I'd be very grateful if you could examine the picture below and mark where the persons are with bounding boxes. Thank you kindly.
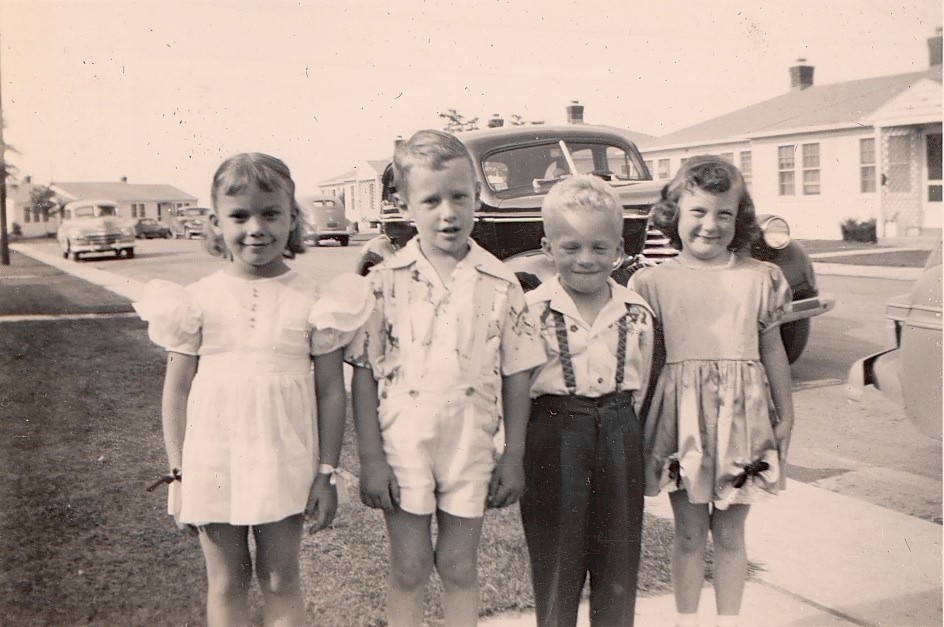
[628,156,793,627]
[146,153,375,627]
[342,129,549,627]
[518,175,656,627]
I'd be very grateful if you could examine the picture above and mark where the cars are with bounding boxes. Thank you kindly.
[133,217,173,241]
[846,232,943,441]
[174,206,214,241]
[352,121,836,367]
[295,194,349,247]
[55,200,136,260]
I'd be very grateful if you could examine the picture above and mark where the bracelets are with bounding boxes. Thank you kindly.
[146,468,182,492]
[318,464,349,485]
